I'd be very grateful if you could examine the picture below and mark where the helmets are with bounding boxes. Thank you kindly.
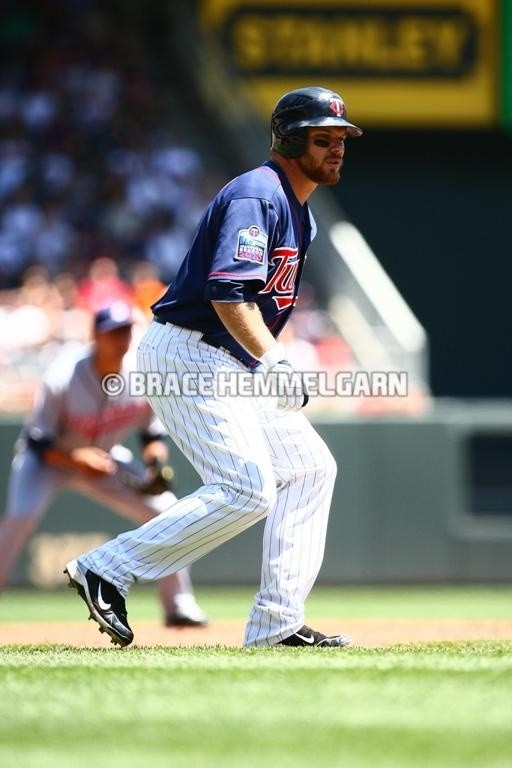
[265,89,366,158]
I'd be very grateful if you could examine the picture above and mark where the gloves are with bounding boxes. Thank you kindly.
[261,342,309,412]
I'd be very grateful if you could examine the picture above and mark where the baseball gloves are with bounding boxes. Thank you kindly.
[131,457,174,495]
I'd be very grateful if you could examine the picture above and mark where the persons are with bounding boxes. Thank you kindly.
[1,300,211,628]
[0,0,432,418]
[61,86,363,651]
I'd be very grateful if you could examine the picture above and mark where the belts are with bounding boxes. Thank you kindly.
[153,313,255,372]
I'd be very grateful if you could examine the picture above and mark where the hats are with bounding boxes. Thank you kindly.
[85,303,136,338]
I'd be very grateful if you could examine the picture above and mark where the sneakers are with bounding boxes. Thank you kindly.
[63,559,133,647]
[273,625,354,646]
[160,610,214,629]
